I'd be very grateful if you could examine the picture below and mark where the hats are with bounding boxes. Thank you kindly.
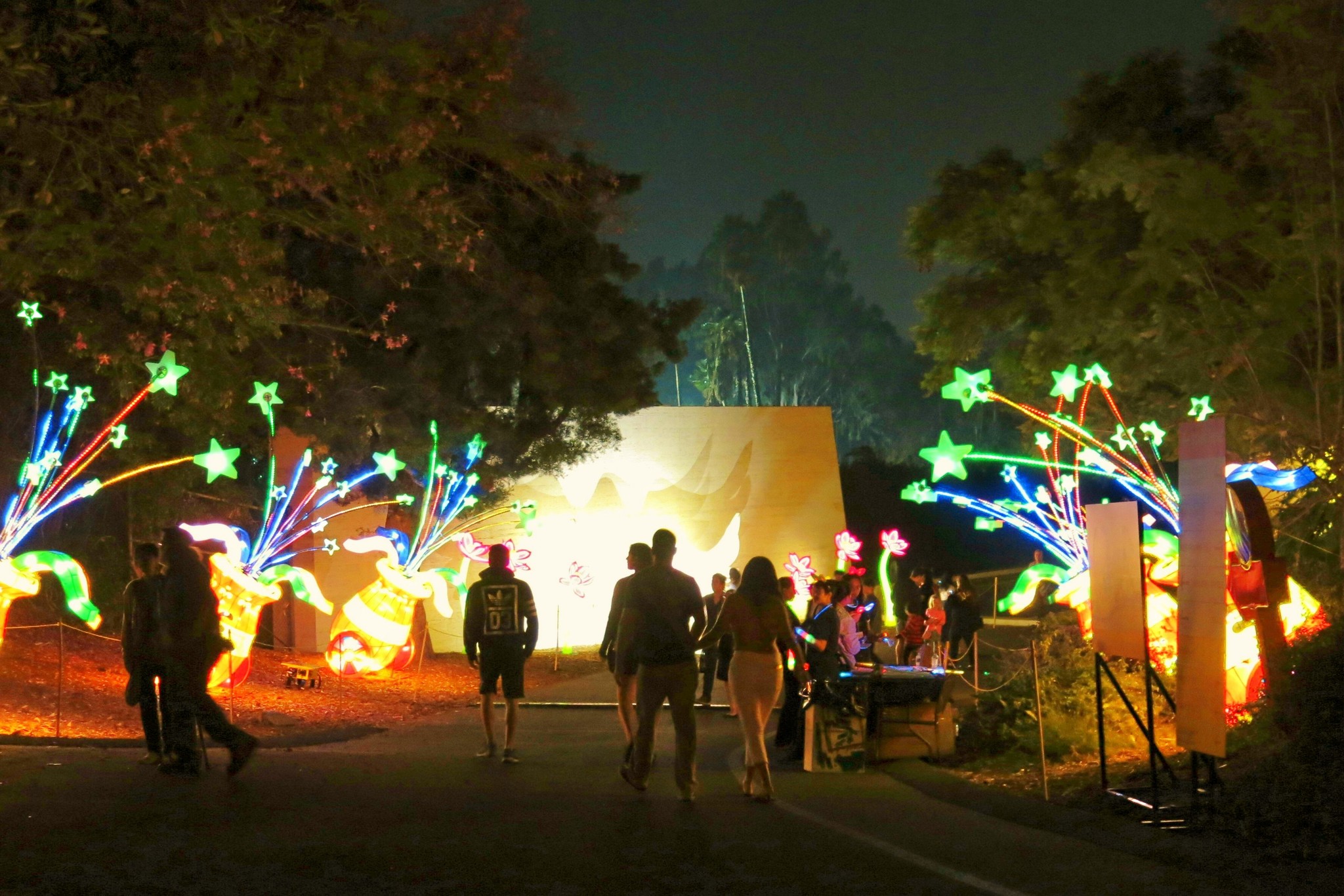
[161,526,195,545]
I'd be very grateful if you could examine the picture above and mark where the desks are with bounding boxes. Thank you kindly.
[840,667,964,760]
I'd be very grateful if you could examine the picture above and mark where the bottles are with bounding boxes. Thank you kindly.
[931,653,939,668]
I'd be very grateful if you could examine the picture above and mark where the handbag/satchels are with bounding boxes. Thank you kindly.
[125,675,143,706]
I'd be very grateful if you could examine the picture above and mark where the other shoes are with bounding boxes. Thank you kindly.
[695,696,712,706]
[226,731,258,777]
[158,749,203,775]
[138,751,157,765]
[618,764,648,790]
[502,748,519,764]
[623,742,633,763]
[476,743,496,757]
[778,746,804,764]
[678,794,695,804]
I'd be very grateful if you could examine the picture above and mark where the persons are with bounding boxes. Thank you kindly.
[599,528,1046,807]
[122,529,258,774]
[463,544,538,762]
